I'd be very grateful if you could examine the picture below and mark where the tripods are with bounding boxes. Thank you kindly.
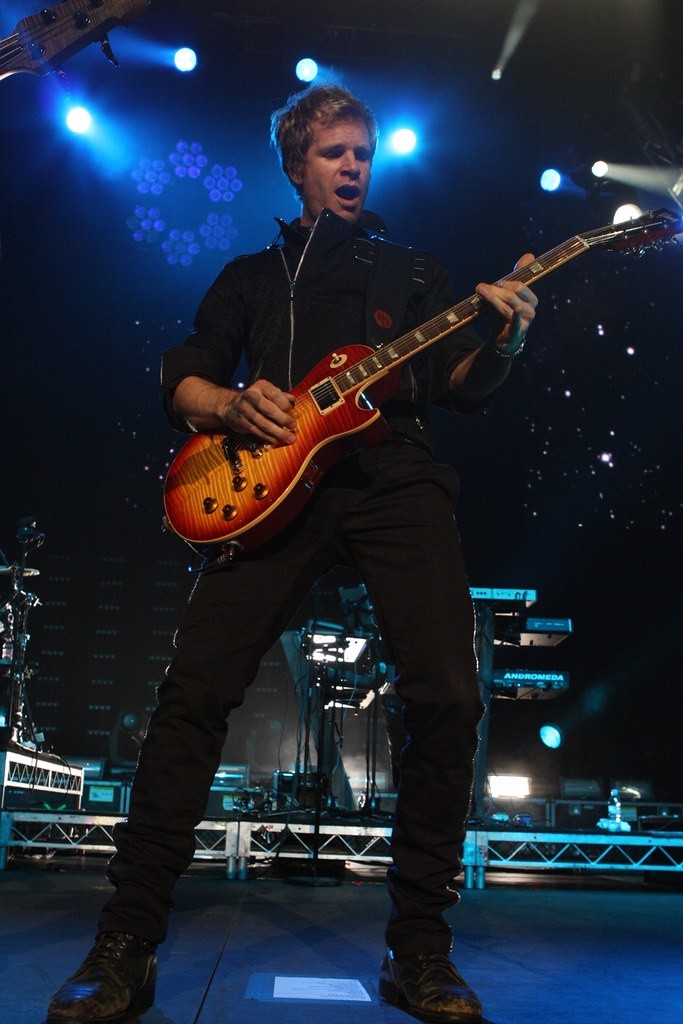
[258,654,397,825]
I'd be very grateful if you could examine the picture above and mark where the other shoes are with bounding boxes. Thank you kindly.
[379,946,483,1022]
[47,933,157,1024]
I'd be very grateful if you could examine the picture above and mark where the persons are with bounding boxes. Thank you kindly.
[45,81,543,1024]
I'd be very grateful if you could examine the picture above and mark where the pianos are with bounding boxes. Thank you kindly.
[492,615,573,648]
[490,668,569,700]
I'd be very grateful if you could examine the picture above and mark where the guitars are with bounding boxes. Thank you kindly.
[162,207,683,559]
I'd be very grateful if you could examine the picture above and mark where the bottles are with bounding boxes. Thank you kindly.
[607,789,621,822]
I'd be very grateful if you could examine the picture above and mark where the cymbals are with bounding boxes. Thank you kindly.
[0,566,40,577]
